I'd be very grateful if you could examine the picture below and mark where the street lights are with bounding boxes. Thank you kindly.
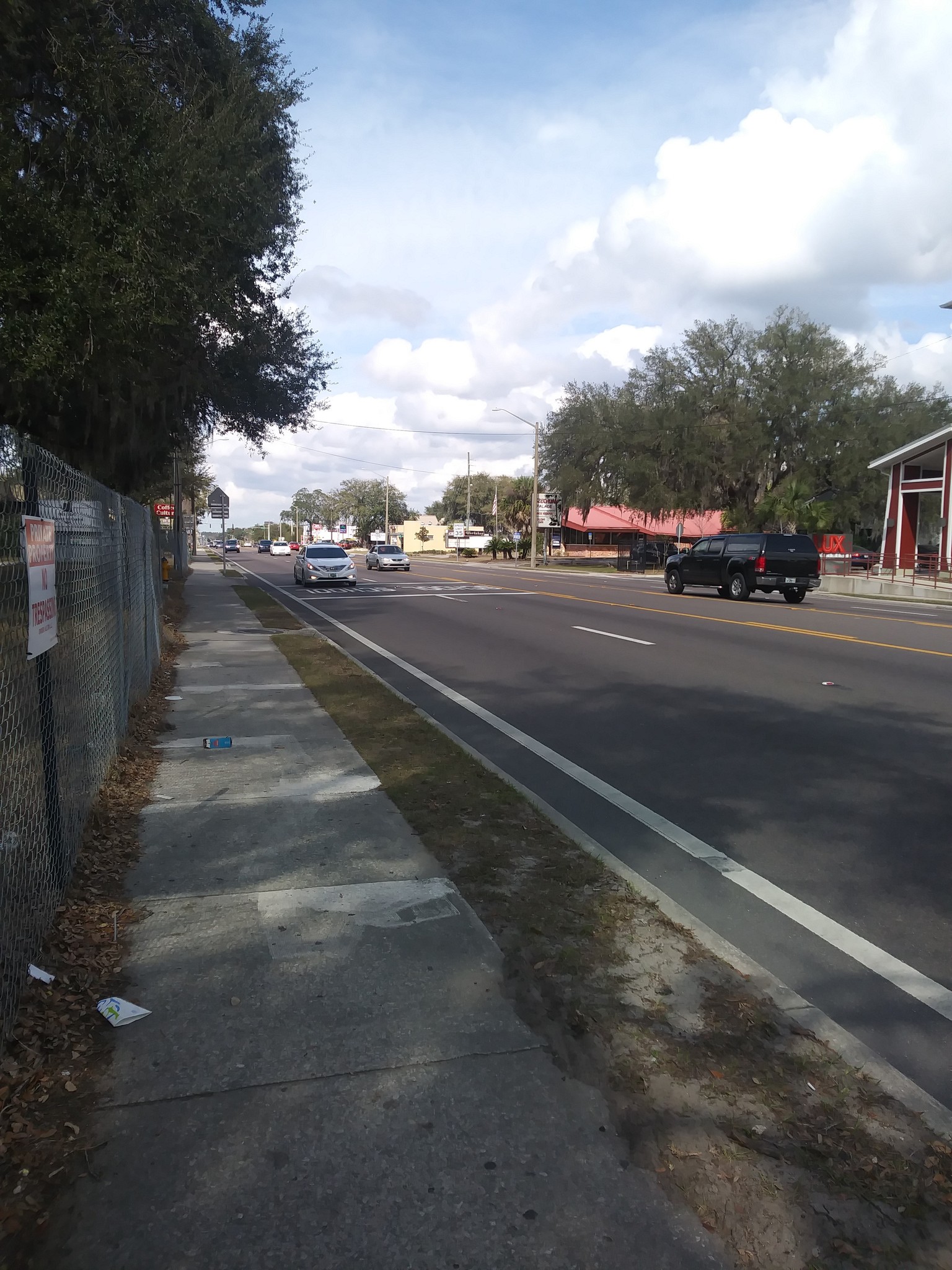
[282,503,298,543]
[492,408,539,567]
[361,468,389,545]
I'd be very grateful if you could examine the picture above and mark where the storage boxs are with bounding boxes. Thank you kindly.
[203,737,233,749]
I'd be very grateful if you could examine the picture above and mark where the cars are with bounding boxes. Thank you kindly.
[244,542,252,547]
[294,544,357,587]
[917,544,939,568]
[348,540,358,547]
[216,541,226,549]
[209,542,213,547]
[270,542,291,556]
[339,540,350,549]
[852,545,878,570]
[303,541,316,545]
[365,544,410,571]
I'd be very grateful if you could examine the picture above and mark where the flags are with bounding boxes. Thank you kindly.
[493,493,498,516]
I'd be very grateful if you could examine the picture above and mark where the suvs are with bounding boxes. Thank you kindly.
[288,542,299,551]
[225,540,240,553]
[631,541,682,565]
[213,540,220,548]
[664,533,822,603]
[257,540,272,553]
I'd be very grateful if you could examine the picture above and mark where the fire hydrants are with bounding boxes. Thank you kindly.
[162,557,170,581]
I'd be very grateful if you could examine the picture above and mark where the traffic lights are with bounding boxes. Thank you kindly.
[252,529,254,532]
[232,529,234,532]
[243,528,244,532]
